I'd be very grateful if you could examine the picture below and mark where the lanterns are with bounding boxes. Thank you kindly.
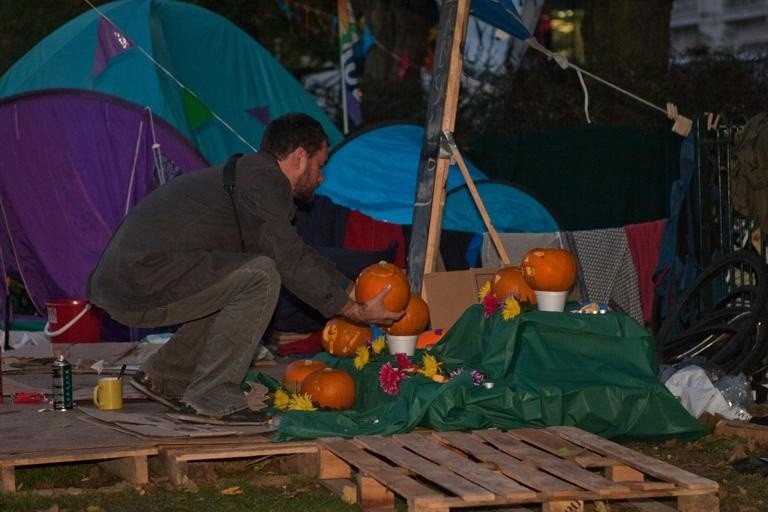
[284,358,327,396]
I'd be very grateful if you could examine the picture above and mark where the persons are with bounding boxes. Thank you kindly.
[80,108,408,428]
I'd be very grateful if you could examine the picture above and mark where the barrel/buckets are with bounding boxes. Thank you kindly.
[43,296,105,343]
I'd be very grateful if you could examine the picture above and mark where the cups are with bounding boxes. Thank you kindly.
[92,377,123,410]
[386,333,418,356]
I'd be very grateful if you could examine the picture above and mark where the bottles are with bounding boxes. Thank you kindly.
[52,353,73,409]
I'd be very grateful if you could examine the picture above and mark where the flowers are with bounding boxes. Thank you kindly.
[354,337,487,399]
[473,278,537,322]
[268,382,320,413]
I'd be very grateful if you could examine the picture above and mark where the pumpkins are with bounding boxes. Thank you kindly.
[284,357,326,387]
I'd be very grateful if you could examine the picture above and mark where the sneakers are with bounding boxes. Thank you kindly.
[130,368,181,413]
[180,403,268,429]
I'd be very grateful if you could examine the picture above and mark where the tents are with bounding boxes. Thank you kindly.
[0,1,564,344]
